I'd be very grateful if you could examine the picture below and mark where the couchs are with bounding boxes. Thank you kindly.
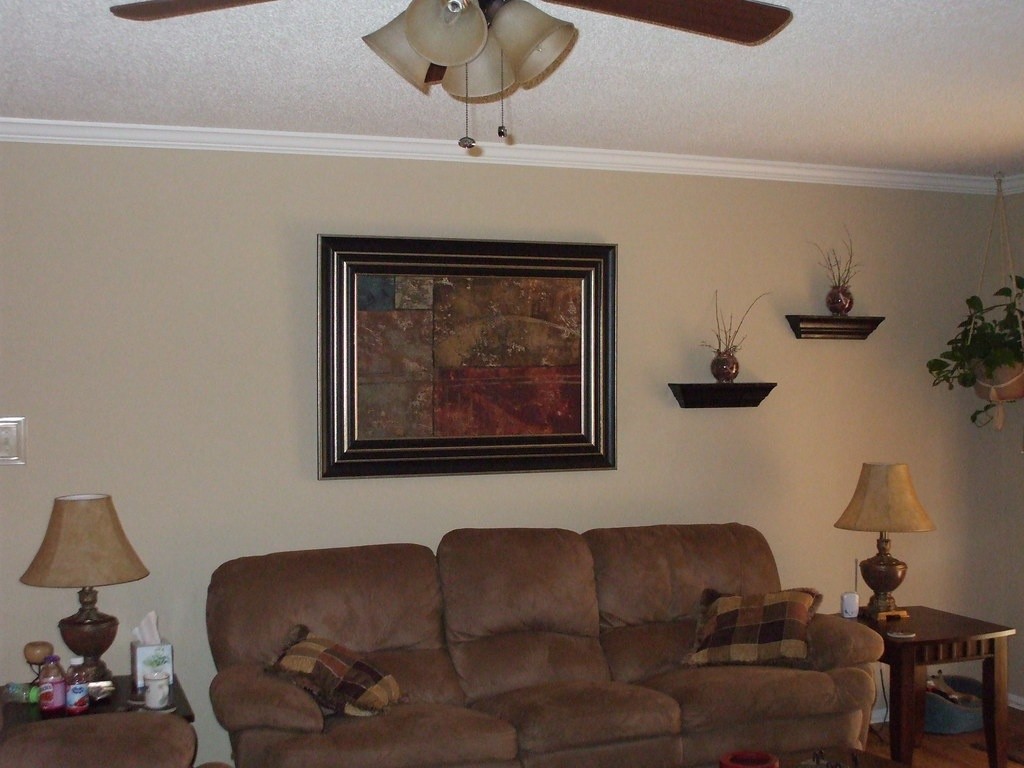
[0,714,196,768]
[205,521,884,768]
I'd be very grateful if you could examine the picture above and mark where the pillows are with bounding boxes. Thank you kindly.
[685,586,822,669]
[264,625,400,717]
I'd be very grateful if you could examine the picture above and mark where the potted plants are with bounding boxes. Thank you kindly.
[926,274,1024,431]
[803,225,861,316]
[700,286,771,383]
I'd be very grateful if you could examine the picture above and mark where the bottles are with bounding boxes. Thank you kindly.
[63,656,89,716]
[2,681,40,705]
[38,656,65,719]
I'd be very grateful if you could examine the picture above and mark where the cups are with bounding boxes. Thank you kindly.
[143,671,171,710]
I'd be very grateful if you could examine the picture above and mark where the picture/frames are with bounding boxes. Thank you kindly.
[316,232,618,481]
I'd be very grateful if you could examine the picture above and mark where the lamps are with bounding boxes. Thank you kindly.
[832,462,937,617]
[359,0,580,150]
[18,493,150,656]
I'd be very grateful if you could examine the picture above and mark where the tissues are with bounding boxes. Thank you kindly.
[130,608,175,688]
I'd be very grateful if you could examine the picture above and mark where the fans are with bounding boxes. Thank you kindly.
[110,0,793,47]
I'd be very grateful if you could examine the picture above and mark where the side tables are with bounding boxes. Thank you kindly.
[0,674,194,722]
[831,605,1017,768]
